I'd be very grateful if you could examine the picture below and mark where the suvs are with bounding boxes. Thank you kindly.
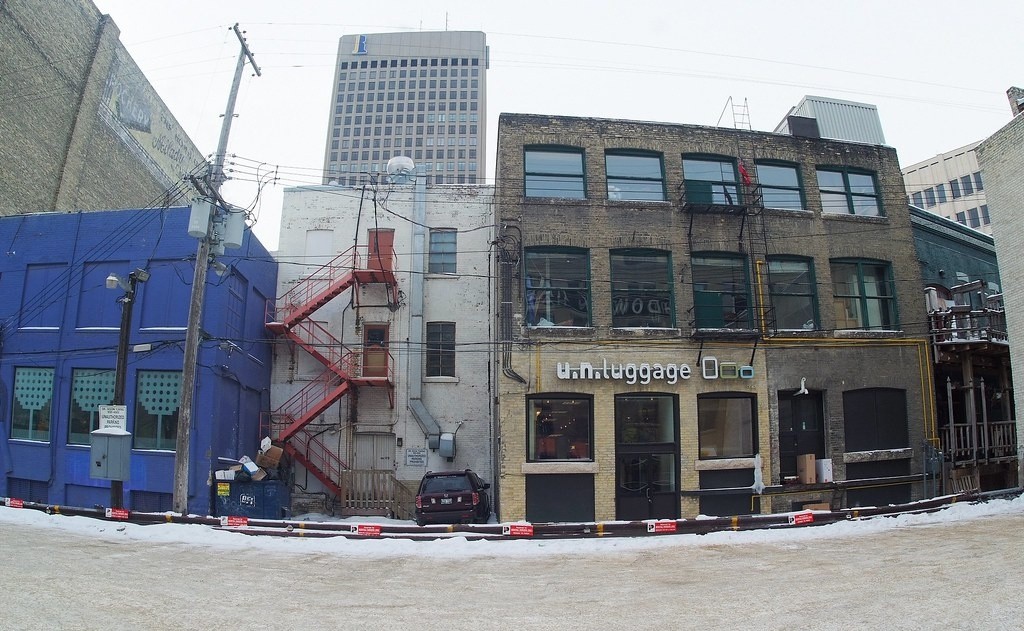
[415,468,491,526]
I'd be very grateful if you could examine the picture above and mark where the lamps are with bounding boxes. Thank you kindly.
[397,437,402,446]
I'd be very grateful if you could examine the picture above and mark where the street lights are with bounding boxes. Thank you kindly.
[105,273,133,509]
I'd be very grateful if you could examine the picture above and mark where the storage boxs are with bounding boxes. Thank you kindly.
[796,453,815,484]
[802,502,830,509]
[256,445,283,468]
[815,458,832,483]
[218,457,238,468]
[250,468,266,480]
[230,465,242,471]
[244,462,258,475]
[240,455,252,463]
[215,470,235,480]
